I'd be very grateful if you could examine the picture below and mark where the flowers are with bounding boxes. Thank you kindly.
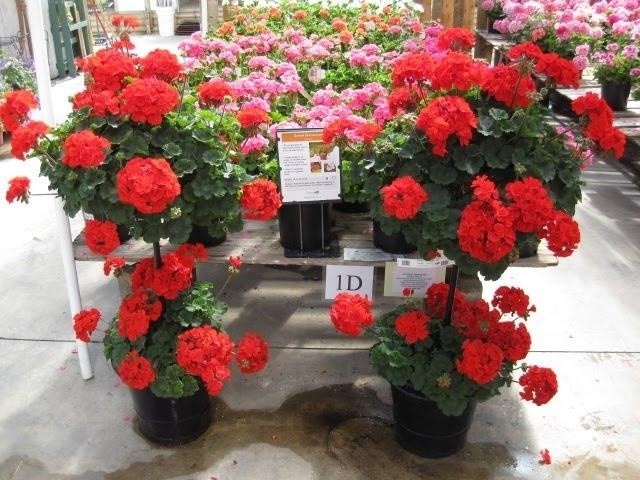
[557,91,626,169]
[181,0,477,221]
[1,47,182,255]
[482,1,640,81]
[110,16,137,50]
[72,242,269,398]
[457,171,582,265]
[478,41,581,110]
[328,286,558,406]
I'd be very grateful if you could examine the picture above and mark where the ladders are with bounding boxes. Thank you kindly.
[48,0,93,80]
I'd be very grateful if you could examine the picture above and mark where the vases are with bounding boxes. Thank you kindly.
[391,380,475,459]
[130,373,212,445]
